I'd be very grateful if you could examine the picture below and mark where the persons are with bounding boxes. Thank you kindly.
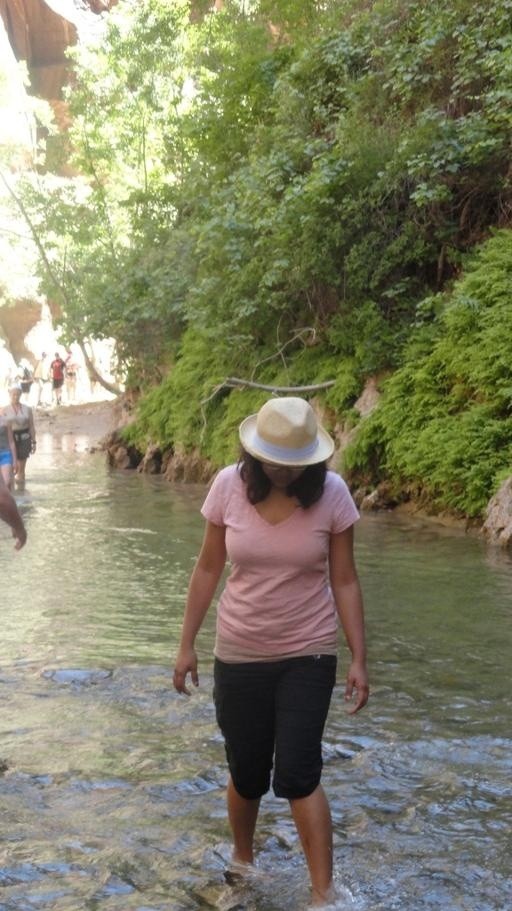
[32,350,49,407]
[0,413,18,490]
[47,353,69,406]
[0,467,28,551]
[64,356,79,401]
[0,383,38,484]
[172,394,371,911]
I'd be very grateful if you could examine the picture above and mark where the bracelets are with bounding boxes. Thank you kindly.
[30,440,36,444]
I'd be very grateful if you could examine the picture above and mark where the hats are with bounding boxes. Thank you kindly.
[238,394,336,466]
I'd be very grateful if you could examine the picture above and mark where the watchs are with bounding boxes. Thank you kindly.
[17,364,31,398]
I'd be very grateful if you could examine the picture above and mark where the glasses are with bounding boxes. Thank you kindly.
[261,463,308,471]
